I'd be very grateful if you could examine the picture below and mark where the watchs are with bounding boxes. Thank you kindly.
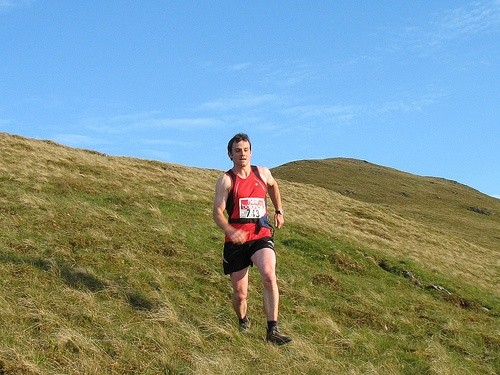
[275,209,284,215]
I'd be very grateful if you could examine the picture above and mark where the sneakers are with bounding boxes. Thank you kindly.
[239,318,248,328]
[266,329,291,345]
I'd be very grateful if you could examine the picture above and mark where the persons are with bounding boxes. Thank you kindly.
[213,132,293,346]
